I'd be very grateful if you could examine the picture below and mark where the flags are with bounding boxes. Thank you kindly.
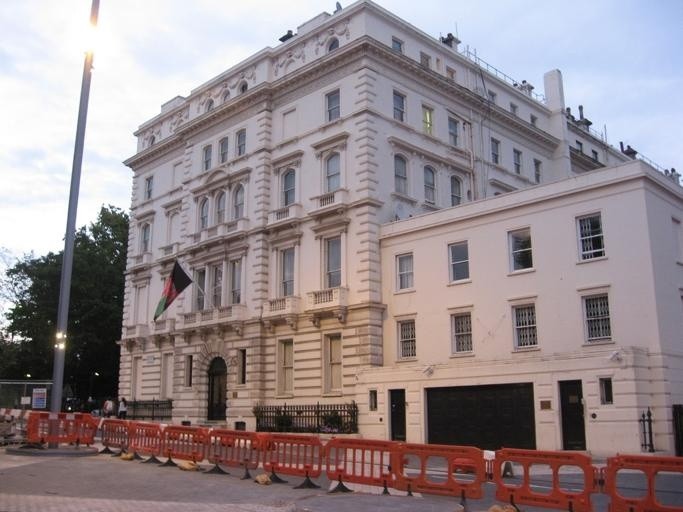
[152,257,193,322]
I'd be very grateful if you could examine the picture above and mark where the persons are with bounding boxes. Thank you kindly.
[117,396,128,420]
[103,396,114,418]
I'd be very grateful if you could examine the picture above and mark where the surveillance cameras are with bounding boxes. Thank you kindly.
[609,350,620,362]
[423,366,433,373]
